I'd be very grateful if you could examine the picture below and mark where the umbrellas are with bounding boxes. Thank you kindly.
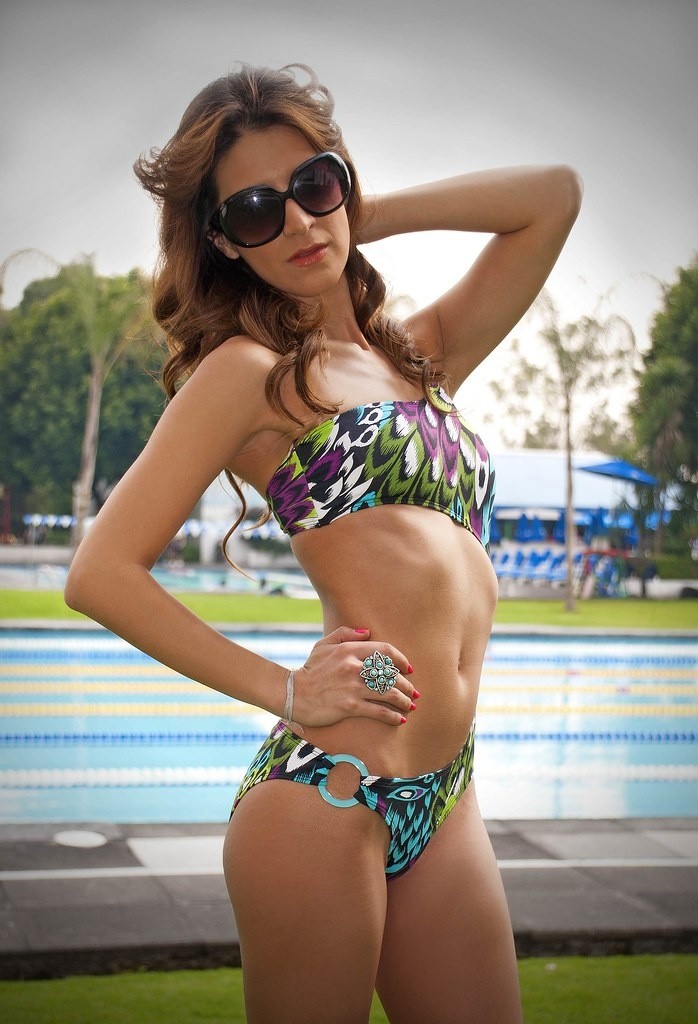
[489,512,607,545]
[577,461,657,486]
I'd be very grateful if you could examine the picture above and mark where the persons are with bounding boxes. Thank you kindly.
[64,64,582,1022]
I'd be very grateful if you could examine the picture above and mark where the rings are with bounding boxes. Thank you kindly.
[361,650,400,693]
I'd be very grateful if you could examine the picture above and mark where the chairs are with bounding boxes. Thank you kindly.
[489,549,657,599]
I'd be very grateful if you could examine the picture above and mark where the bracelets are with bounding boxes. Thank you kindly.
[284,668,294,720]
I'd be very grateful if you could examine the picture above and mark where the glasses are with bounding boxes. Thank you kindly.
[207,152,352,248]
